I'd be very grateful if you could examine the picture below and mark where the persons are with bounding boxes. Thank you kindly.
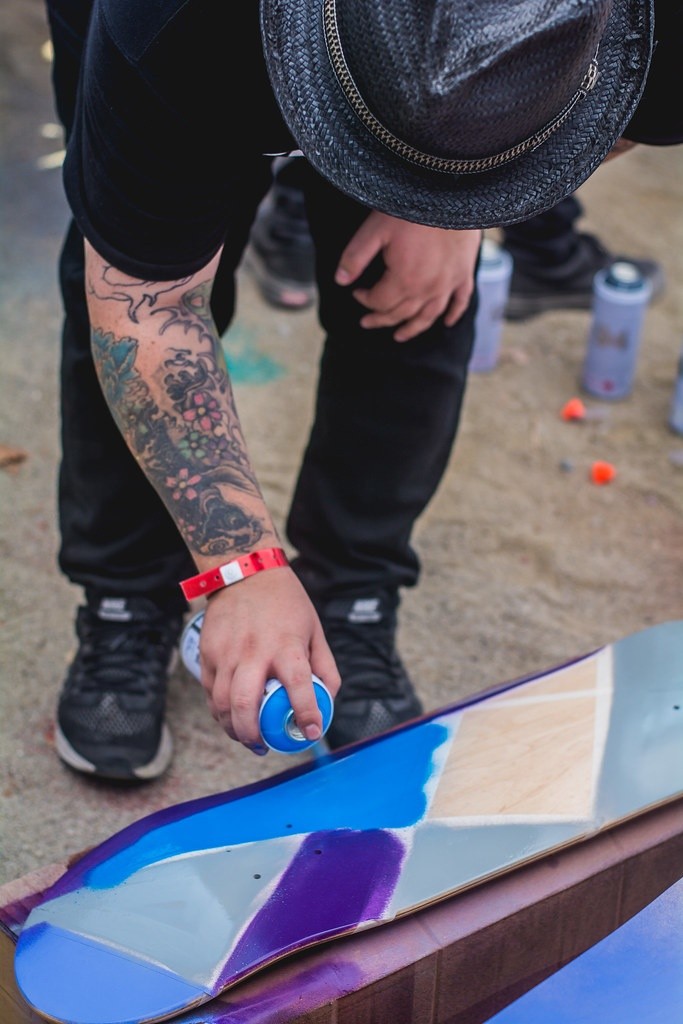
[245,0,682,320]
[44,1,655,793]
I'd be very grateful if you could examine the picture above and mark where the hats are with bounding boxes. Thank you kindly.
[260,0,655,231]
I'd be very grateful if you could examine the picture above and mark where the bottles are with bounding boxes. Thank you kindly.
[180,608,335,755]
[579,261,652,397]
[466,238,514,372]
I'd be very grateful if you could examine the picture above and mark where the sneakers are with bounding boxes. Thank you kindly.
[247,208,316,307]
[311,598,423,754]
[52,595,184,786]
[501,236,663,316]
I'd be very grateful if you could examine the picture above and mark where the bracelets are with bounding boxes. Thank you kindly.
[179,546,289,602]
